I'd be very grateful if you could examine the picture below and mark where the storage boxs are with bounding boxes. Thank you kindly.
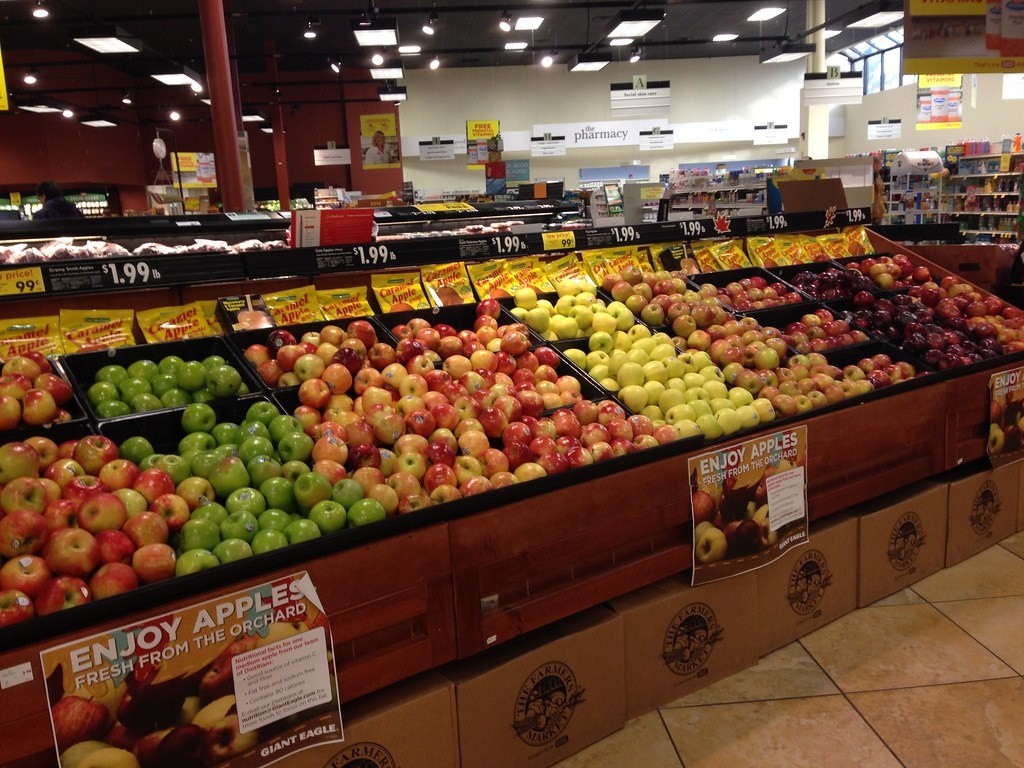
[0,242,1024,768]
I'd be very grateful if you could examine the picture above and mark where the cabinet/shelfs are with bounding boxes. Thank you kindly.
[595,152,1024,238]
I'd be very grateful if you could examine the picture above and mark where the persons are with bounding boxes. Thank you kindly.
[31,181,86,219]
[366,131,393,165]
[872,156,887,225]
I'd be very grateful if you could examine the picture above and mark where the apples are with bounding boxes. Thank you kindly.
[52,634,260,768]
[0,252,1023,627]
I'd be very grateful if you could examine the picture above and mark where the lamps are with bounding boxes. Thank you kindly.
[241,0,905,134]
[16,25,203,133]
